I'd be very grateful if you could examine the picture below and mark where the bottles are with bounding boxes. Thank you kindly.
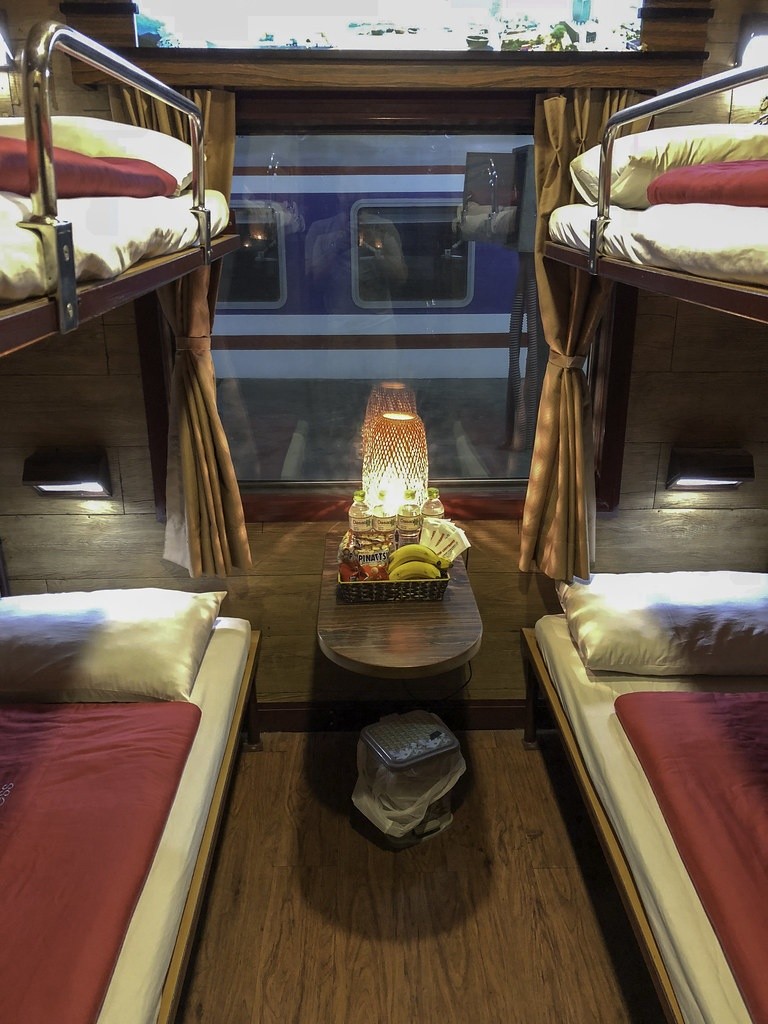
[373,490,397,553]
[422,488,445,526]
[349,491,372,538]
[397,489,421,548]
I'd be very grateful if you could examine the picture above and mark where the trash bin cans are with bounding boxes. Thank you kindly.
[358,709,460,848]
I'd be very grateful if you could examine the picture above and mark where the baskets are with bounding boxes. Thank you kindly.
[337,569,450,602]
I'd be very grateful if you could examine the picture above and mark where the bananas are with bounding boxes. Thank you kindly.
[388,544,453,580]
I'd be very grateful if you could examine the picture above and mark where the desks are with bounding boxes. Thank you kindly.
[316,531,483,679]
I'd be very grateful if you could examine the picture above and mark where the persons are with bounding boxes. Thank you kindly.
[304,173,408,423]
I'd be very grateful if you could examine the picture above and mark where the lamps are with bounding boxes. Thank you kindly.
[22,443,112,498]
[666,443,756,491]
[359,382,416,460]
[736,12,768,65]
[361,413,430,513]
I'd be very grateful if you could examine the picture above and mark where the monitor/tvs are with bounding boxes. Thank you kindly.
[130,0,647,57]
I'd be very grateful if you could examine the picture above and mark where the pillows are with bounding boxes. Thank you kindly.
[0,587,228,704]
[556,570,768,676]
[570,122,768,210]
[0,116,207,195]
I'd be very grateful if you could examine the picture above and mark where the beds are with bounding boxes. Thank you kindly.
[1,617,263,1024]
[0,21,242,356]
[544,63,768,327]
[519,613,768,1024]
